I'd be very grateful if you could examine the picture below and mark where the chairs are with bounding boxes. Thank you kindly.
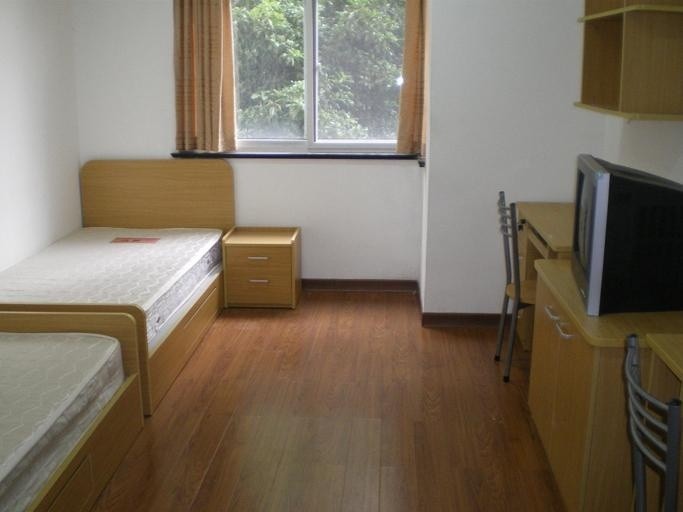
[624,334,682,512]
[493,191,538,383]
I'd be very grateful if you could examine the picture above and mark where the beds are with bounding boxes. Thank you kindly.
[0,311,144,512]
[0,158,235,417]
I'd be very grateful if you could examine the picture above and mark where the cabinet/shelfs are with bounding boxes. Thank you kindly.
[573,0,683,121]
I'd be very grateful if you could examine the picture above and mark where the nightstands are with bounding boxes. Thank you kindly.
[222,225,301,310]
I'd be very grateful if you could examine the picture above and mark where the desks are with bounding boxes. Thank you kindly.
[526,259,683,512]
[515,200,575,354]
[632,332,683,512]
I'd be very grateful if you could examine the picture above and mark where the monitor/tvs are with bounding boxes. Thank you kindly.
[570,152,683,318]
